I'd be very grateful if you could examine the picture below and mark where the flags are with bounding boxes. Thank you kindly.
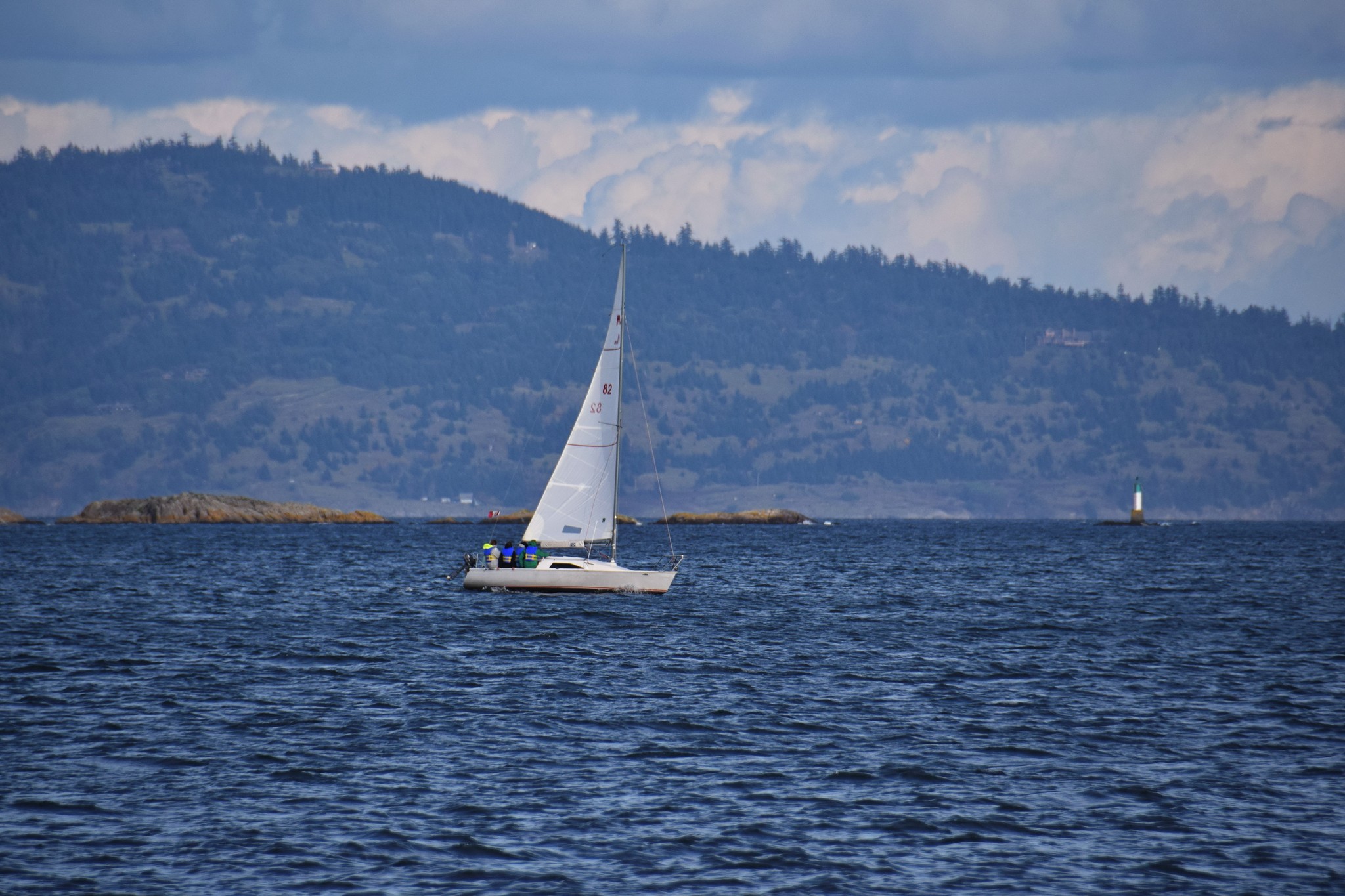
[488,511,501,518]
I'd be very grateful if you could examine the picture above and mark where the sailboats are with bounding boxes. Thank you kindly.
[447,242,681,595]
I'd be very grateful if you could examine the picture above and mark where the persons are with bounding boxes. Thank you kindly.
[519,539,551,568]
[483,539,501,569]
[513,542,528,568]
[497,539,516,570]
